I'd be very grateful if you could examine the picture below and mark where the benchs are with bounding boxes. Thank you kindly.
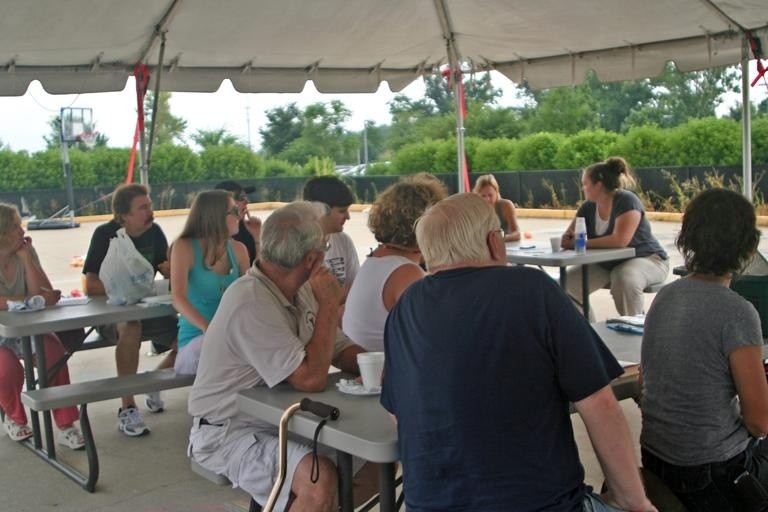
[0,331,152,422]
[602,280,665,325]
[188,456,231,487]
[21,367,197,494]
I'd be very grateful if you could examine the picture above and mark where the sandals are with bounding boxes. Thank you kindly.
[56,429,85,449]
[4,415,33,440]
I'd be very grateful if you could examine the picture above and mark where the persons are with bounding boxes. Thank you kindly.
[302,178,362,330]
[186,202,367,512]
[378,192,659,511]
[0,203,86,449]
[557,158,671,326]
[82,185,178,436]
[641,187,768,512]
[472,174,520,241]
[170,191,250,374]
[340,174,449,353]
[214,179,262,267]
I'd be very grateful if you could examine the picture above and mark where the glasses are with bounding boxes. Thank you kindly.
[317,241,333,252]
[226,206,240,218]
[496,228,507,236]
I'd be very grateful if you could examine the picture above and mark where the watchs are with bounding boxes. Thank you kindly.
[23,293,31,307]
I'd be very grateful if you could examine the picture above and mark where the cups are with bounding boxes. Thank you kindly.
[551,237,561,254]
[356,351,385,390]
[154,280,170,296]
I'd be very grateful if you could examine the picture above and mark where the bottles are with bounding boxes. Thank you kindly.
[575,218,587,257]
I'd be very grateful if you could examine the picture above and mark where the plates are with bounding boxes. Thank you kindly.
[337,381,382,397]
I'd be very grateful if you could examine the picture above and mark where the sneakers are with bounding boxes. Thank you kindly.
[119,406,150,437]
[146,393,163,411]
